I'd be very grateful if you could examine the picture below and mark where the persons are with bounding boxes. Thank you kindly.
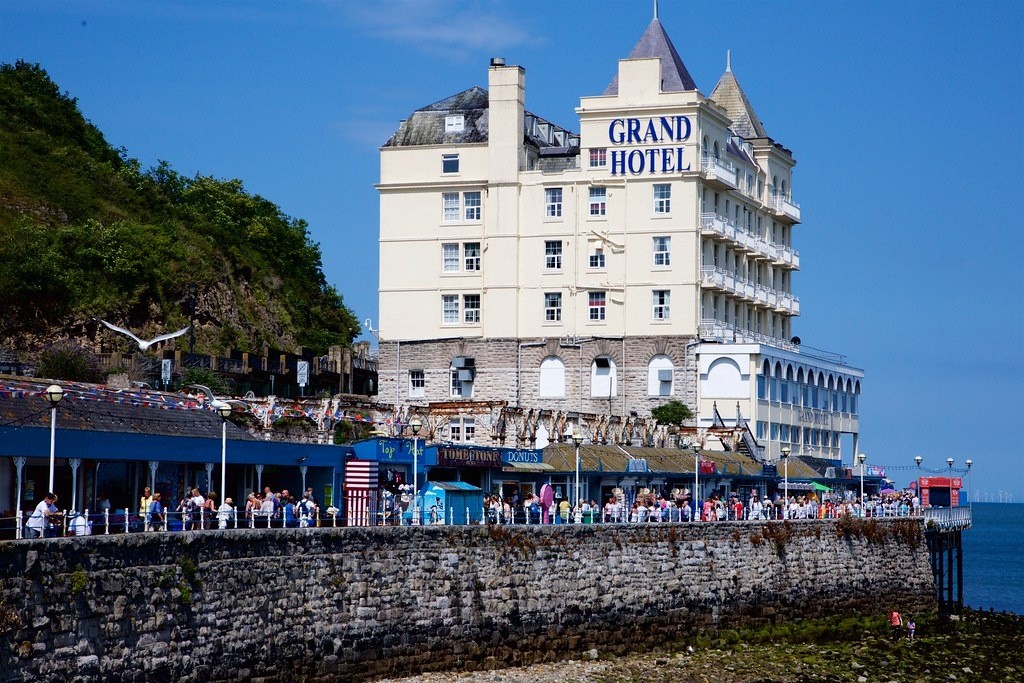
[477,486,923,524]
[886,608,903,645]
[24,484,324,539]
[906,616,916,642]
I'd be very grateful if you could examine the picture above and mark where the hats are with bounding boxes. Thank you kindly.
[224,498,233,504]
[68,509,80,518]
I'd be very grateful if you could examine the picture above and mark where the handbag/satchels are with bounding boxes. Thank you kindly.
[151,501,162,522]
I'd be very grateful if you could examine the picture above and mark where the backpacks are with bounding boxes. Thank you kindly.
[297,499,309,518]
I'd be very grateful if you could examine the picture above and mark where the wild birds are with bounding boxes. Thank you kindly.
[91,315,193,352]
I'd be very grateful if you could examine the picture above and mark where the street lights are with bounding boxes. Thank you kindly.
[965,459,973,522]
[572,433,584,523]
[692,441,702,522]
[408,419,423,524]
[914,455,923,516]
[215,402,232,505]
[41,384,66,494]
[857,453,866,518]
[946,457,955,522]
[780,446,790,519]
[188,281,198,363]
